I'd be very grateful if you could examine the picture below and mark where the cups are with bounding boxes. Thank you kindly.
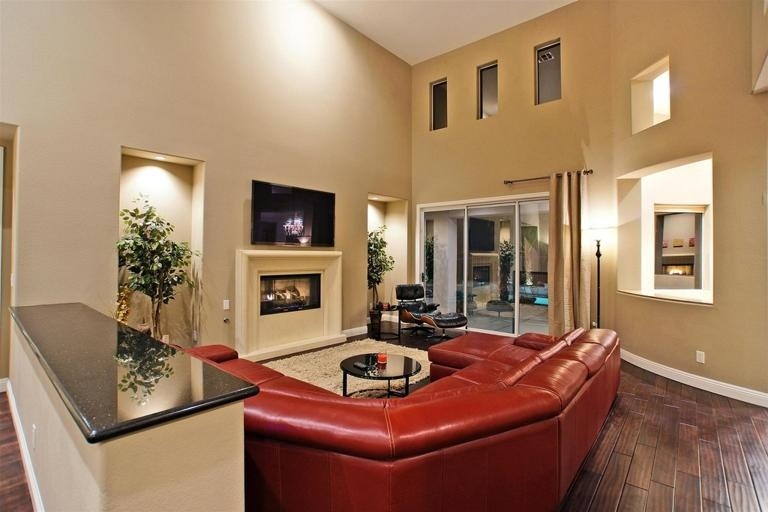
[378,349,387,364]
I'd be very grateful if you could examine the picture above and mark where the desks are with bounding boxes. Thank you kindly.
[7,300,261,511]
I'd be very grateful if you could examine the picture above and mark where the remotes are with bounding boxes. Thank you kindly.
[353,362,367,370]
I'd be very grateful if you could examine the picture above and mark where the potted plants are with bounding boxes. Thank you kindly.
[367,224,395,332]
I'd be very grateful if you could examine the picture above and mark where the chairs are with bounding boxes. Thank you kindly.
[396,282,469,343]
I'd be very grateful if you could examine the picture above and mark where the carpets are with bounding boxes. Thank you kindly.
[262,337,432,398]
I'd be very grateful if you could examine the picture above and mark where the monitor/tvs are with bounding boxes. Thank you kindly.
[250,178,335,248]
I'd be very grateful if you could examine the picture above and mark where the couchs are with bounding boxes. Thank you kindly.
[169,324,623,511]
